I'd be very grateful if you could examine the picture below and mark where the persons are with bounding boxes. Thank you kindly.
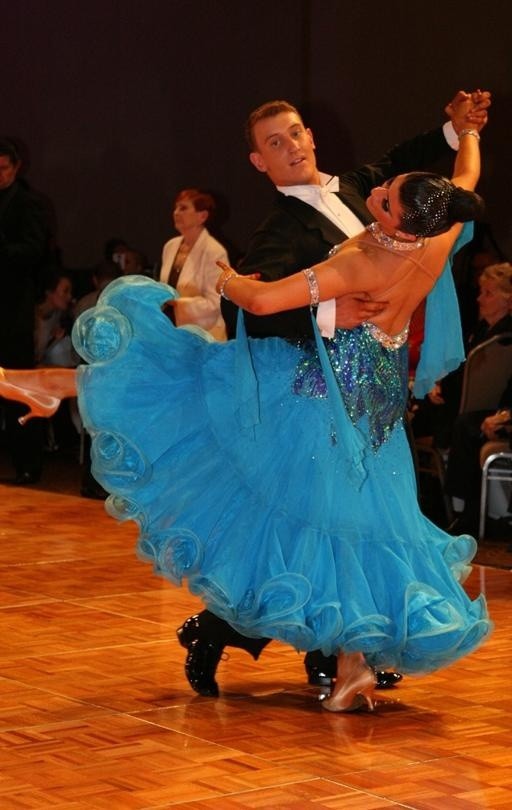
[434,399,512,536]
[31,237,145,450]
[1,89,490,716]
[412,262,512,535]
[175,89,490,697]
[157,189,230,344]
[1,144,49,478]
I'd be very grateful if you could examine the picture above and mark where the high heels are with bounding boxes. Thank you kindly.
[321,663,378,713]
[1,367,60,426]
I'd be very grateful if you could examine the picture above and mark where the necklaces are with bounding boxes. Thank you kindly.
[369,221,425,252]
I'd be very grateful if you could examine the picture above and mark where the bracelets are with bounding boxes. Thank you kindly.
[219,269,234,304]
[457,126,480,141]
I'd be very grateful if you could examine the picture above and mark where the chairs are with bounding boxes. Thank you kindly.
[413,335,511,538]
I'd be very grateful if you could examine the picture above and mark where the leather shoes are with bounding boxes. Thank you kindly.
[176,614,230,697]
[304,649,402,688]
